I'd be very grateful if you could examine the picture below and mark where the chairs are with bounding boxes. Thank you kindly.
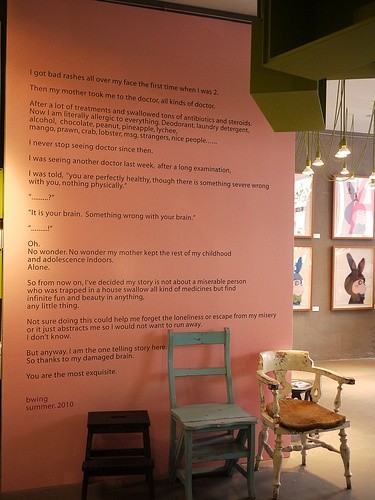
[167,327,259,500]
[254,350,356,500]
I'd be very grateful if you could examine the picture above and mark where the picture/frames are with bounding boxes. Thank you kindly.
[331,244,375,311]
[331,173,375,241]
[294,170,316,239]
[293,243,315,312]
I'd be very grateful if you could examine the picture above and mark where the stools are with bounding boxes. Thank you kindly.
[80,410,156,500]
[291,378,319,442]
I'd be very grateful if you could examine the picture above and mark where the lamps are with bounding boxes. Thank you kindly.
[296,79,375,190]
